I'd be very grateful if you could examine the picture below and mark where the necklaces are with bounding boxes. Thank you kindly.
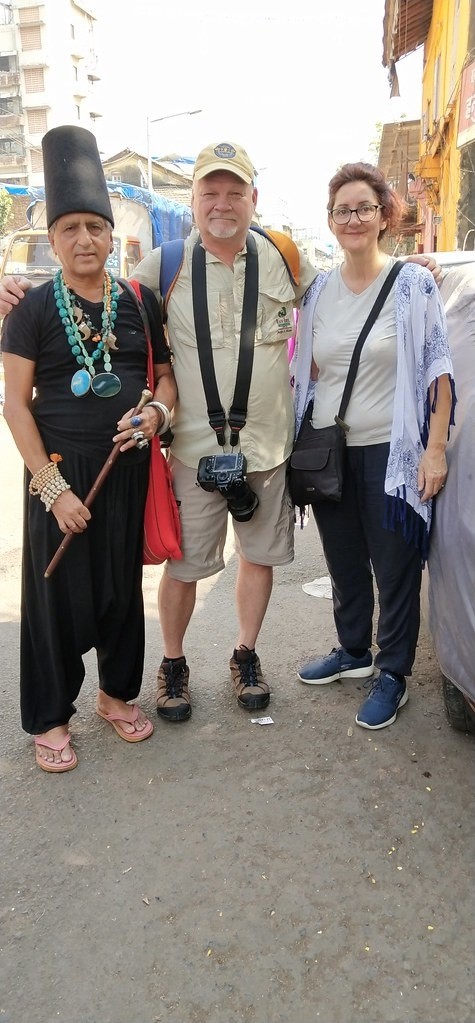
[54,268,119,376]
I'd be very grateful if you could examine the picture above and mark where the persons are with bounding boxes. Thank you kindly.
[0,143,443,722]
[290,161,455,729]
[0,124,176,773]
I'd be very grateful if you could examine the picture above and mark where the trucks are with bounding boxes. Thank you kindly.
[1,192,193,289]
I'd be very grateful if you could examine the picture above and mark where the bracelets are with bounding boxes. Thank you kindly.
[145,402,170,435]
[29,454,71,512]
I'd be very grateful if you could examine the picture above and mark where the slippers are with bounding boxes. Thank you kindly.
[34,732,78,772]
[96,705,153,743]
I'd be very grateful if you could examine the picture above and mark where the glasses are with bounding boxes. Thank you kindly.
[330,204,383,225]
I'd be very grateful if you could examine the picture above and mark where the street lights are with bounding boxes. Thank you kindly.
[147,109,202,189]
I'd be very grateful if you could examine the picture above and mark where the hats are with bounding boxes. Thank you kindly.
[41,125,114,229]
[192,142,255,184]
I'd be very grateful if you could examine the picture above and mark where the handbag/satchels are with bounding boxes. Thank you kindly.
[289,425,343,506]
[143,435,183,564]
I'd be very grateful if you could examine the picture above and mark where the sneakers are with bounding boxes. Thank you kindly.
[157,659,190,722]
[297,647,373,685]
[355,670,409,729]
[229,644,269,709]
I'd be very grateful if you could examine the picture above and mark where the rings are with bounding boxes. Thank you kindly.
[136,439,148,449]
[130,416,143,428]
[132,427,145,441]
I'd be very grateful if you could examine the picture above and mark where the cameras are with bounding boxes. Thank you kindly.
[198,452,259,522]
